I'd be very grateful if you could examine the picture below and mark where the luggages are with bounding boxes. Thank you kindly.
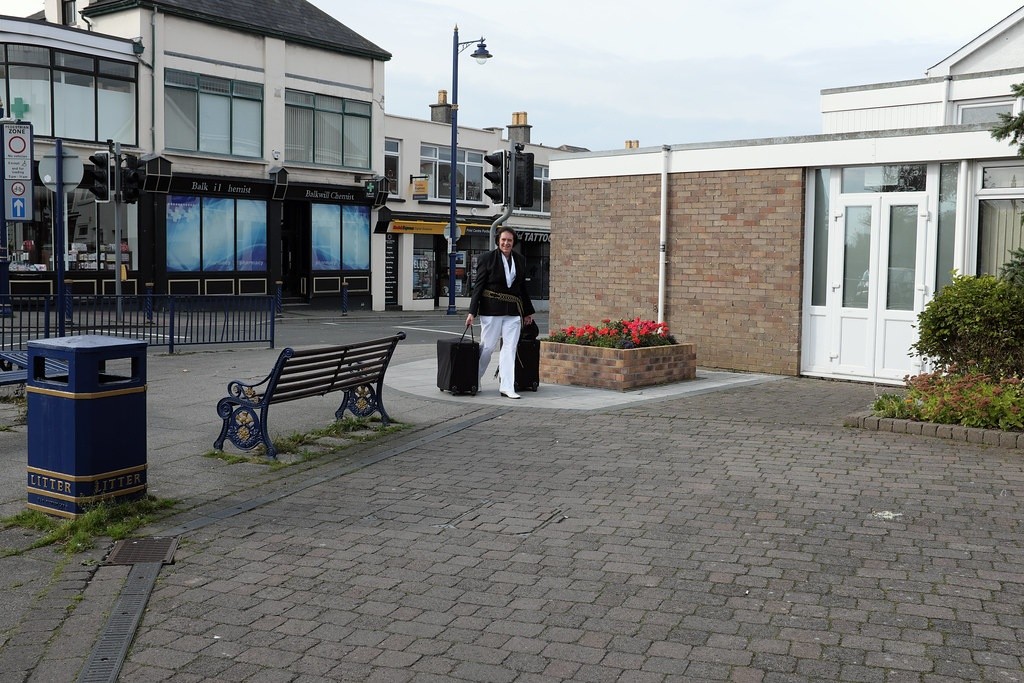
[500,338,540,392]
[437,324,480,396]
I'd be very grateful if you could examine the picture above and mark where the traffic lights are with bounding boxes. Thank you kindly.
[125,153,147,204]
[483,150,506,206]
[89,151,111,203]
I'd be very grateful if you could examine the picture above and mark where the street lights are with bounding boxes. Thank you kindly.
[445,24,492,316]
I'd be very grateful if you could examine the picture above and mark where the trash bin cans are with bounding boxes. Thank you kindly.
[24,335,149,520]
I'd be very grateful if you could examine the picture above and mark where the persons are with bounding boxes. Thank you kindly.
[465,227,536,400]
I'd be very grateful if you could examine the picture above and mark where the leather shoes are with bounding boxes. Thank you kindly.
[501,391,520,399]
[478,387,482,392]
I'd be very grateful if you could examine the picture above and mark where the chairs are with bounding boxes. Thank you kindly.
[212,331,407,463]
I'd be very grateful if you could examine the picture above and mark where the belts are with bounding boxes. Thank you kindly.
[483,290,525,328]
